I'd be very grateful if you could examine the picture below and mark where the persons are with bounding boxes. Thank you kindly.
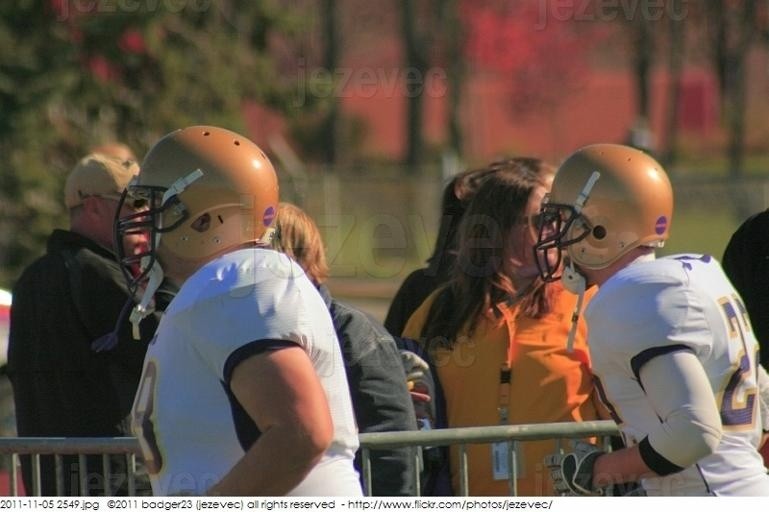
[7,125,769,495]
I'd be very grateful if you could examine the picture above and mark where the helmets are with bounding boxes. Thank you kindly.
[113,126,280,298]
[531,144,675,283]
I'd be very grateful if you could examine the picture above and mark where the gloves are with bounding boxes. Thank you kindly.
[544,439,608,497]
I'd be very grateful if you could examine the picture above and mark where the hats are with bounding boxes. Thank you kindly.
[64,154,141,212]
